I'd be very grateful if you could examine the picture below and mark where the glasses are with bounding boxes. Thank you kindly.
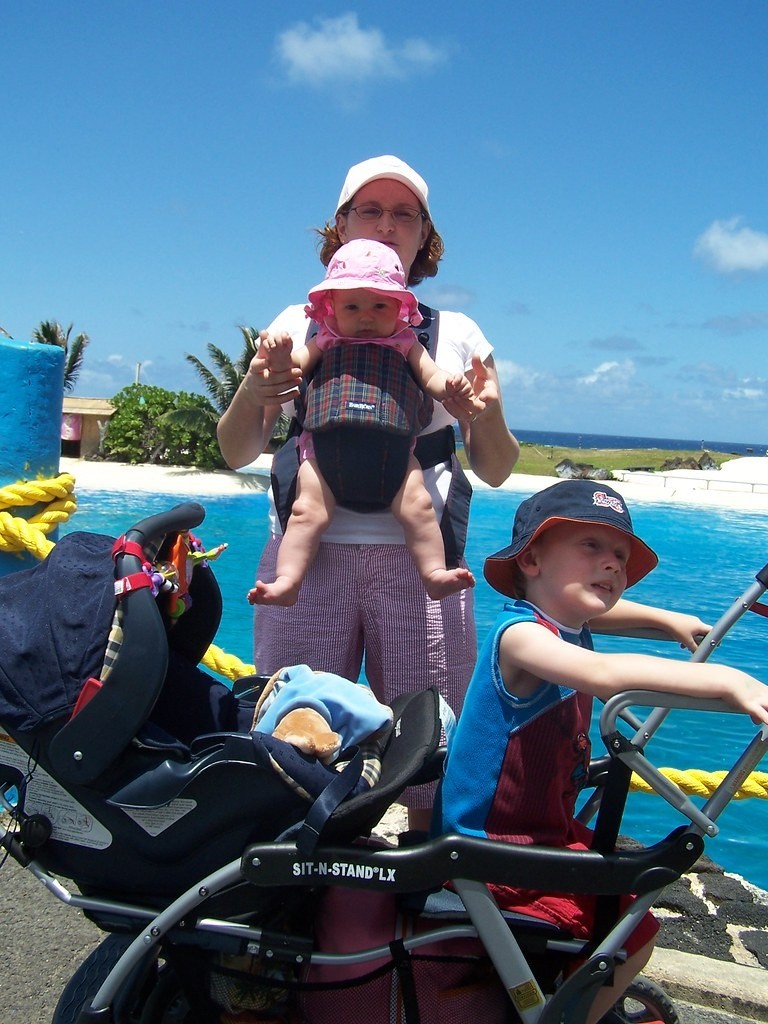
[337,201,429,223]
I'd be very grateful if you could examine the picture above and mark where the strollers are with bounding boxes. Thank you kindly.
[2,505,768,1024]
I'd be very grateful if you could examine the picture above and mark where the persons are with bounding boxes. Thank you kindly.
[431,478,767,1023]
[246,237,487,606]
[216,153,520,835]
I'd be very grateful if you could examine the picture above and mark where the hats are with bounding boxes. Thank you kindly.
[301,237,426,328]
[334,155,431,219]
[479,475,659,599]
